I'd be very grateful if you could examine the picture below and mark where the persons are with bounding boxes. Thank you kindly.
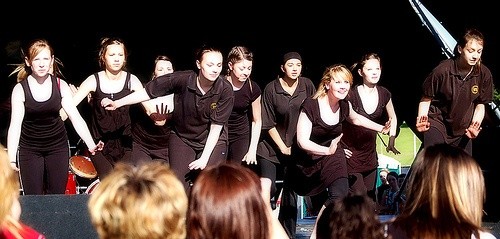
[59,37,170,182]
[310,195,384,239]
[349,52,401,202]
[86,160,189,239]
[279,64,394,239]
[257,52,316,209]
[381,142,497,239]
[6,40,104,195]
[0,145,45,239]
[222,46,263,165]
[416,27,493,156]
[376,168,407,215]
[142,56,174,130]
[101,43,235,198]
[185,162,290,239]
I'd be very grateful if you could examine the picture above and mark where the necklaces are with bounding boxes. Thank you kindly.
[104,71,124,98]
